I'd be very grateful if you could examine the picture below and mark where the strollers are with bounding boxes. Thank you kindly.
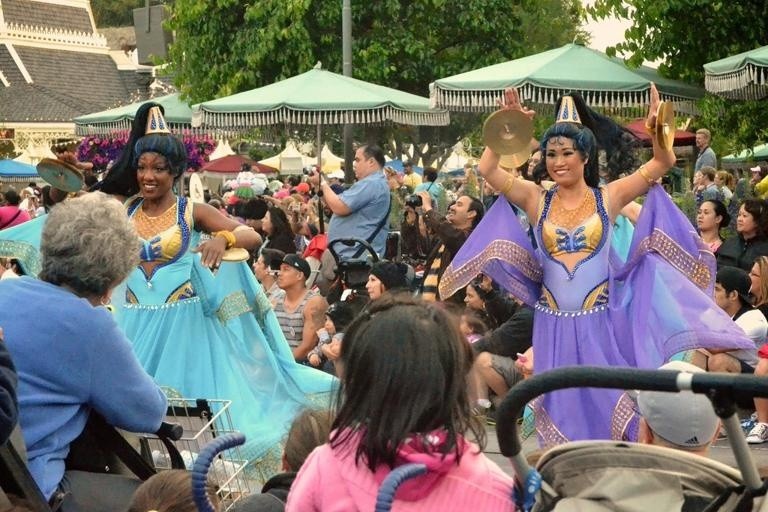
[496,365,768,511]
[328,238,444,295]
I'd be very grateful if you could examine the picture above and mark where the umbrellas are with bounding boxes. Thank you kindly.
[721,142,768,176]
[191,60,451,167]
[439,82,755,453]
[622,119,696,148]
[0,156,40,186]
[73,92,193,137]
[702,45,768,100]
[429,36,705,117]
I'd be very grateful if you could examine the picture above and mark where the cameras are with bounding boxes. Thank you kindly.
[405,195,436,207]
[303,165,321,175]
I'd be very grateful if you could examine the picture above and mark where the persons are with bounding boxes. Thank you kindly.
[517,161,698,442]
[189,163,206,204]
[0,189,31,232]
[258,207,296,274]
[365,261,408,301]
[0,190,169,512]
[402,160,421,192]
[27,183,37,197]
[698,166,719,203]
[713,171,734,207]
[308,300,354,380]
[414,167,443,214]
[707,266,768,441]
[241,198,268,243]
[253,248,288,311]
[416,189,485,307]
[593,83,594,88]
[125,469,227,512]
[716,201,768,274]
[750,165,768,202]
[463,282,500,331]
[240,162,327,238]
[306,143,392,262]
[328,169,352,192]
[745,343,768,444]
[633,361,723,459]
[95,102,346,485]
[527,147,544,181]
[227,409,336,512]
[696,198,731,261]
[236,186,257,219]
[19,187,38,219]
[469,264,534,425]
[748,256,768,323]
[460,308,499,417]
[283,294,519,512]
[694,128,717,174]
[463,162,478,196]
[270,254,328,372]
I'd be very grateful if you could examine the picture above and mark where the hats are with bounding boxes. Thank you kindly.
[750,165,761,172]
[245,200,267,220]
[370,261,408,286]
[6,192,20,205]
[403,159,414,167]
[717,266,755,306]
[283,254,312,278]
[326,302,354,332]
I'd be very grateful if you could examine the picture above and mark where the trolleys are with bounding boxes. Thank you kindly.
[192,432,425,512]
[0,387,250,512]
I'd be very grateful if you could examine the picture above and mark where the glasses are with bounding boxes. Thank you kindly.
[748,270,764,280]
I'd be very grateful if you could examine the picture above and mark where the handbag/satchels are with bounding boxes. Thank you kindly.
[321,193,392,280]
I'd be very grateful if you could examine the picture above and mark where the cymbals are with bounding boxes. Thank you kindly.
[36,159,85,193]
[656,99,674,152]
[498,144,532,169]
[221,246,250,262]
[482,110,534,155]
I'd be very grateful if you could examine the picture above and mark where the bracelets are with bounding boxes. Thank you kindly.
[213,229,237,249]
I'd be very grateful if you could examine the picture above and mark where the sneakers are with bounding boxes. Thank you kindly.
[743,415,757,429]
[471,401,496,418]
[625,361,719,447]
[746,423,768,443]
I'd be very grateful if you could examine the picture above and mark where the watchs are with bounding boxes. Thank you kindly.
[316,179,328,188]
